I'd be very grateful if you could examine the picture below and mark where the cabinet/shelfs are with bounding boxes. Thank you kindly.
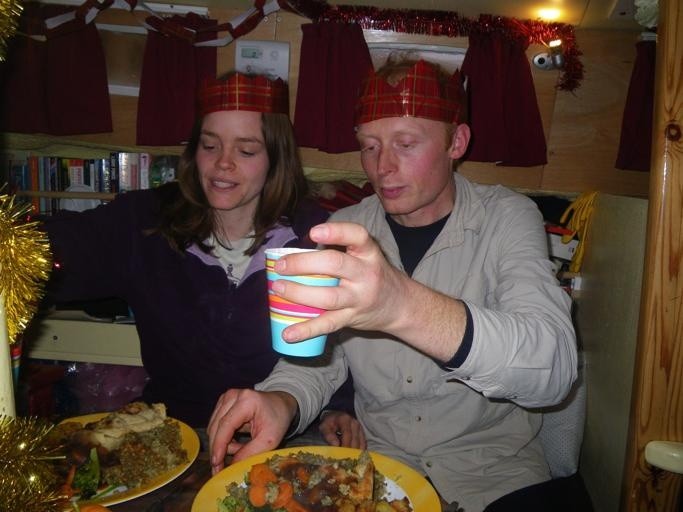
[0,142,191,368]
[304,168,648,512]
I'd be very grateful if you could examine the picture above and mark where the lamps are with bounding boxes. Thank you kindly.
[533,33,563,70]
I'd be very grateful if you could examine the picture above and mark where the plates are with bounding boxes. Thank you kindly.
[47,411,199,510]
[189,445,441,511]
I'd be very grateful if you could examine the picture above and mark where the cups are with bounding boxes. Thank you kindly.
[263,247,336,358]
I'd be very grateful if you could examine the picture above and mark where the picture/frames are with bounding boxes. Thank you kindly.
[364,42,468,84]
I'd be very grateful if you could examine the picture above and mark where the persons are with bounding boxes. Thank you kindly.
[202,49,592,511]
[33,70,368,449]
